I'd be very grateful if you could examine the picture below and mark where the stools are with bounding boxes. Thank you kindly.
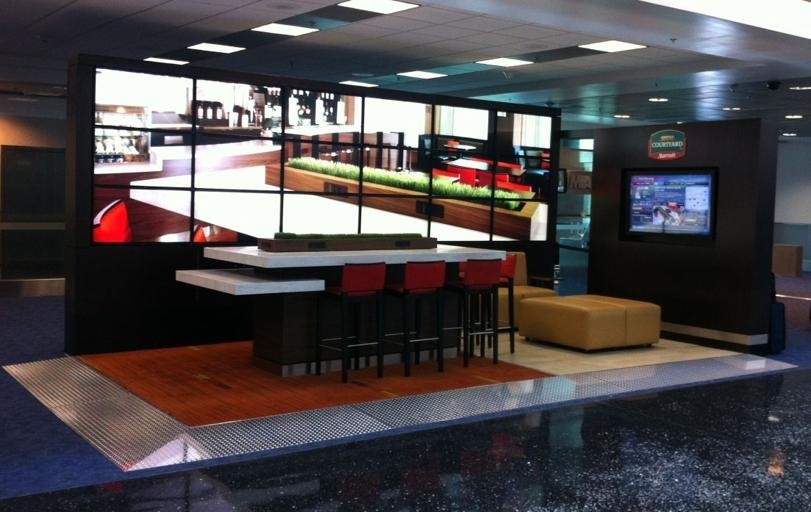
[522,295,662,354]
[466,250,559,329]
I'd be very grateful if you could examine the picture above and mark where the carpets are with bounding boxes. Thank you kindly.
[78,338,554,432]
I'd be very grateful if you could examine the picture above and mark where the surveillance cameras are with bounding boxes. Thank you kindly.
[766,81,779,91]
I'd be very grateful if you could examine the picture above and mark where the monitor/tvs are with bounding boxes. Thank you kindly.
[558,169,568,193]
[628,174,710,235]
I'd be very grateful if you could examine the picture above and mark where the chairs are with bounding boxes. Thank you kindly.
[307,264,385,381]
[443,258,500,367]
[500,253,515,353]
[387,261,446,378]
